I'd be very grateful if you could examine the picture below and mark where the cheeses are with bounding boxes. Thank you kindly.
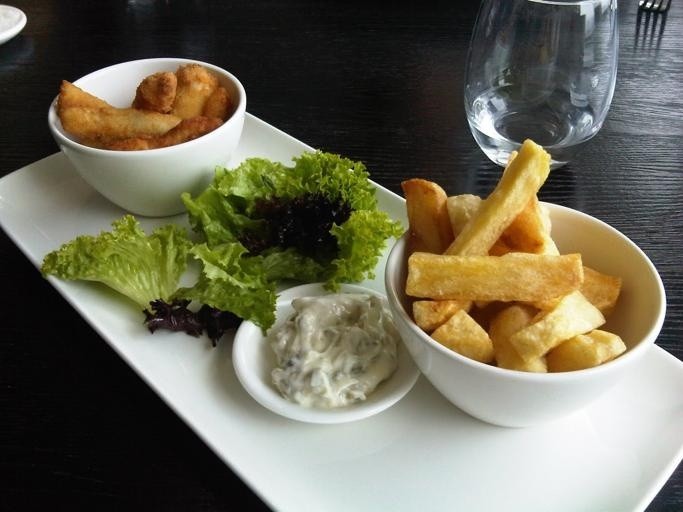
[270,294,398,408]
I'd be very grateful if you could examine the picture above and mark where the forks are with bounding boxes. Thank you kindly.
[634,8,667,38]
[637,1,672,13]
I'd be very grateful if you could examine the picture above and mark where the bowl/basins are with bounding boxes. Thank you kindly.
[382,201,667,430]
[47,57,248,217]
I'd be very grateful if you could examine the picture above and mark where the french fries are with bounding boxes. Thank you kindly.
[401,137,628,374]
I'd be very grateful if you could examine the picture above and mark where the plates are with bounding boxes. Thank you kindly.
[0,113,683,512]
[0,3,28,44]
[230,281,421,424]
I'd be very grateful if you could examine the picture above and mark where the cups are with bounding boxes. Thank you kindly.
[459,0,621,171]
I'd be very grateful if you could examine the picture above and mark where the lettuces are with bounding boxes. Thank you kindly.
[41,148,405,335]
